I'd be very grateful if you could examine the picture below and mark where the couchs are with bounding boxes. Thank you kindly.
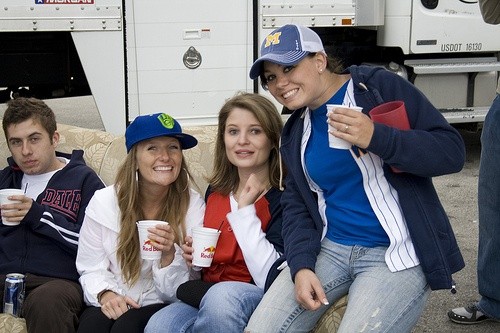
[1,121,350,333]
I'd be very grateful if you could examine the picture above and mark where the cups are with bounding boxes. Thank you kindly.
[0,189,27,227]
[326,104,362,149]
[137,220,168,261]
[368,101,411,173]
[190,226,222,268]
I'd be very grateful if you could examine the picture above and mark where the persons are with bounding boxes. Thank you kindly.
[447,0,500,328]
[235,23,468,333]
[72,112,207,333]
[0,95,108,333]
[141,91,290,333]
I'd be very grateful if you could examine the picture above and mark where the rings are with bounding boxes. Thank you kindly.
[343,124,350,133]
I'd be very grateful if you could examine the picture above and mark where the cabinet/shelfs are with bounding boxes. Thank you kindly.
[124,1,255,130]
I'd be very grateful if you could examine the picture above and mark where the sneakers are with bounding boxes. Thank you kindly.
[448,302,499,324]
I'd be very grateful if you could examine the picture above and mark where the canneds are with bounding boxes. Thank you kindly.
[4,273,27,316]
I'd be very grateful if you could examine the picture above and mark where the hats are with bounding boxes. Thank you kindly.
[249,24,327,79]
[125,113,198,154]
